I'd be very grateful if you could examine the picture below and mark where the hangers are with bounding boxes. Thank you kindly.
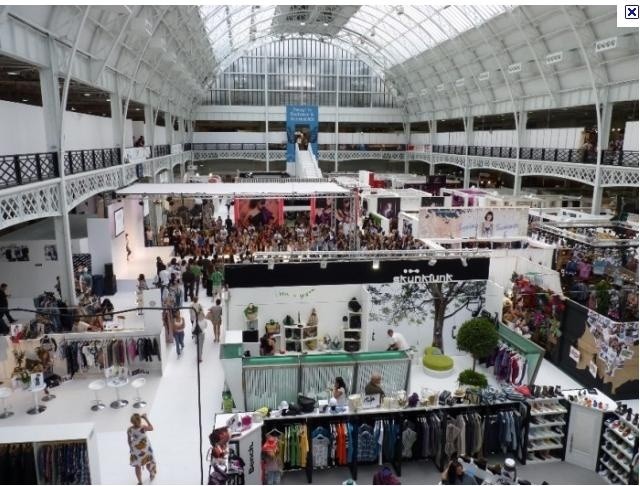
[265,406,522,437]
[1,441,87,456]
[60,334,158,347]
[496,336,526,361]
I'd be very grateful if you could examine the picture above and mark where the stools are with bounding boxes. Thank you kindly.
[0,374,147,418]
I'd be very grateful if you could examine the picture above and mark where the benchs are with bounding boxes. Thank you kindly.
[421,344,456,379]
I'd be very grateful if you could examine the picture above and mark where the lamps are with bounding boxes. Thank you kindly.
[264,251,469,271]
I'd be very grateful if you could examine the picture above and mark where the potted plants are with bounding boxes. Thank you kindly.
[451,314,502,394]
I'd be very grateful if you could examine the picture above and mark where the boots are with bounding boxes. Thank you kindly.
[529,385,536,399]
[620,404,628,416]
[626,407,632,421]
[549,386,557,397]
[555,385,564,396]
[633,413,639,425]
[542,386,552,398]
[615,402,622,413]
[536,385,545,398]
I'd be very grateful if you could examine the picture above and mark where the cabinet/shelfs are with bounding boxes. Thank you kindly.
[594,407,639,485]
[342,328,362,353]
[346,309,363,330]
[0,422,103,485]
[523,394,570,466]
[283,322,319,354]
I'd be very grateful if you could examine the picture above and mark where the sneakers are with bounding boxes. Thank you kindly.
[527,401,566,461]
[599,417,634,484]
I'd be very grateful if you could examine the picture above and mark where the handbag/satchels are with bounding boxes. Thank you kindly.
[286,329,291,337]
[296,343,301,352]
[244,302,259,319]
[304,328,317,337]
[344,331,361,340]
[283,315,294,325]
[308,309,318,325]
[265,320,281,334]
[348,297,362,312]
[344,342,360,352]
[287,342,295,351]
[293,329,300,339]
[303,340,317,350]
[349,315,362,328]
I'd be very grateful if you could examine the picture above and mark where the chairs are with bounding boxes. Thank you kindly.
[112,315,125,331]
